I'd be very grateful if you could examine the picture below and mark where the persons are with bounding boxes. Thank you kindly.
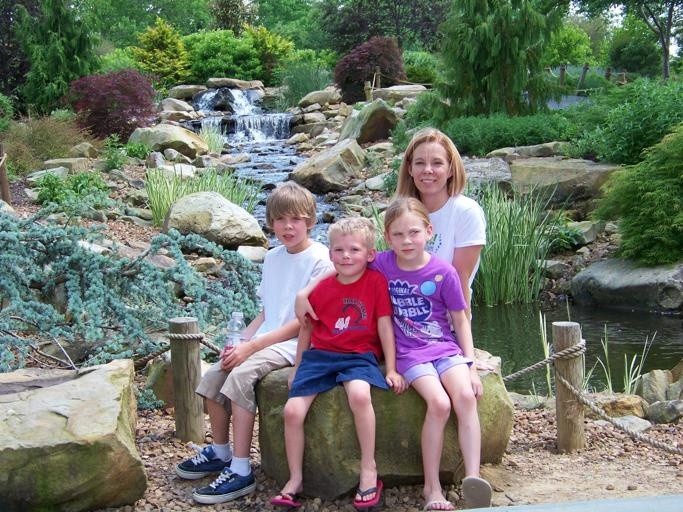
[175,185,335,505]
[294,196,493,511]
[393,128,494,371]
[270,217,409,508]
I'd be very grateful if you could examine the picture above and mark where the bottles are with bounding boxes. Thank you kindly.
[224,311,247,353]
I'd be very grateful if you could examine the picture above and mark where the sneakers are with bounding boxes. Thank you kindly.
[192,468,257,504]
[175,445,233,480]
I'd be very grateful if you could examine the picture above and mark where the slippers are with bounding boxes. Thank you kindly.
[423,501,455,512]
[353,480,383,508]
[271,493,302,507]
[463,477,491,509]
